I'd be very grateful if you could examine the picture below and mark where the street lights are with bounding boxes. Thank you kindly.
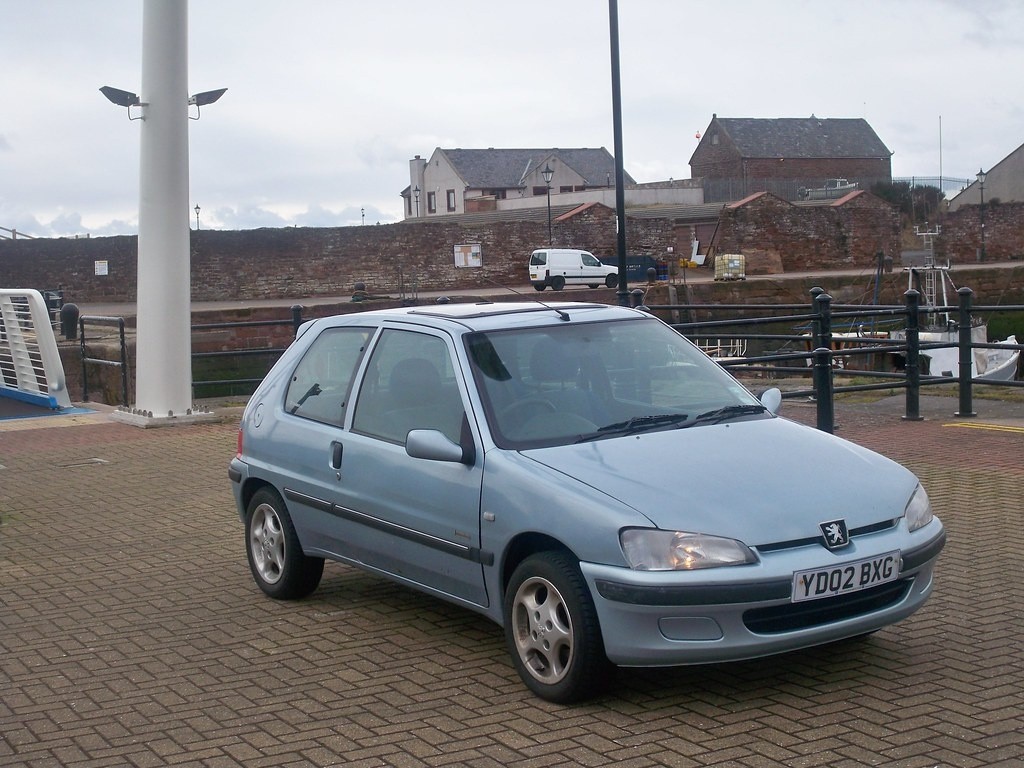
[540,164,554,246]
[361,208,364,226]
[194,205,201,231]
[975,167,987,265]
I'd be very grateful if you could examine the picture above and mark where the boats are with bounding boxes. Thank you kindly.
[792,219,1021,383]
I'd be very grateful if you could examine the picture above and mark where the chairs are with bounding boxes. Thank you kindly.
[533,336,593,422]
[382,357,442,433]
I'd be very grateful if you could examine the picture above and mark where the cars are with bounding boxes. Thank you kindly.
[228,303,947,709]
[45,291,61,308]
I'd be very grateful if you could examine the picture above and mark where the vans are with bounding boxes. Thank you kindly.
[529,248,620,291]
